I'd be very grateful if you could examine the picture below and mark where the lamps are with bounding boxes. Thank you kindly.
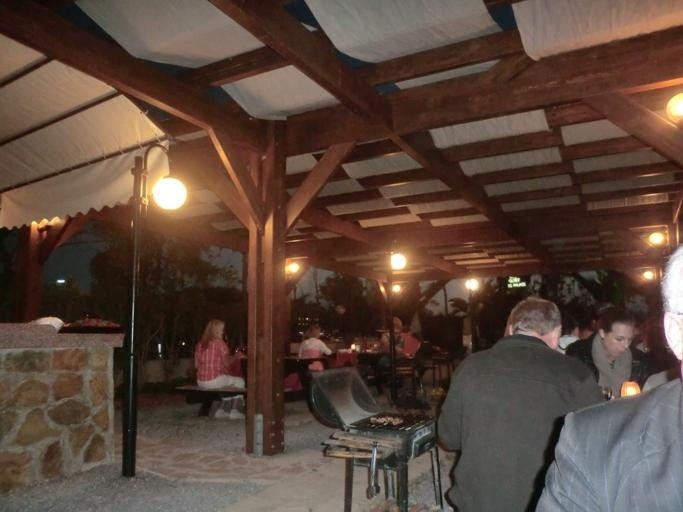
[620,381,640,398]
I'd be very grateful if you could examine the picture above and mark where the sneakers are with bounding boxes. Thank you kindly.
[213,408,246,421]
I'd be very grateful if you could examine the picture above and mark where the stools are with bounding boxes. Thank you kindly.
[431,355,455,388]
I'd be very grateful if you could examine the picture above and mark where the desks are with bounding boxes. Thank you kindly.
[233,350,384,405]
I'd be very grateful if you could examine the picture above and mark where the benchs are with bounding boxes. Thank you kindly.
[177,385,246,417]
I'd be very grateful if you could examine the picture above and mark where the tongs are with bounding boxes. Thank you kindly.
[366,440,381,500]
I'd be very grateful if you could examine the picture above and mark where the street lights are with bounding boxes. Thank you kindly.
[121,143,187,477]
[387,241,406,405]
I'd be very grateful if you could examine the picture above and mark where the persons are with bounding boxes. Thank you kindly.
[376,315,426,392]
[330,304,355,351]
[191,319,246,421]
[297,325,332,371]
